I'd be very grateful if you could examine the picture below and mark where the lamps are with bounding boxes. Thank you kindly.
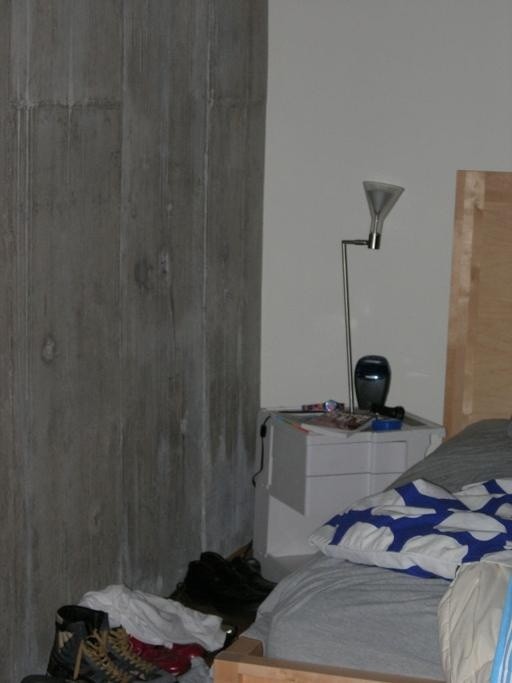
[342,180,406,413]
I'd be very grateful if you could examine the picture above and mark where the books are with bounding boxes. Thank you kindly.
[300,410,376,439]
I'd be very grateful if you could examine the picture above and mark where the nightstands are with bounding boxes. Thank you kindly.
[254,408,446,595]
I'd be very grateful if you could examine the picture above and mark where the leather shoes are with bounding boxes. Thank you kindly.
[167,641,204,662]
[199,552,278,591]
[183,561,269,613]
[129,633,190,675]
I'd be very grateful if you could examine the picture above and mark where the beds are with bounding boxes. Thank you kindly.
[207,169,512,683]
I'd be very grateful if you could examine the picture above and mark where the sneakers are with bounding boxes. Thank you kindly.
[107,624,177,683]
[46,604,149,683]
